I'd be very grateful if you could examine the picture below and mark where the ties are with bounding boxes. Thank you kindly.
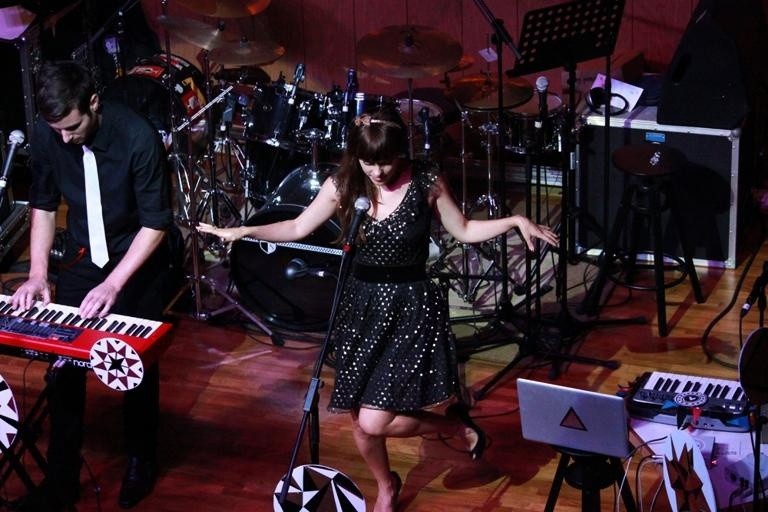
[81,144,110,269]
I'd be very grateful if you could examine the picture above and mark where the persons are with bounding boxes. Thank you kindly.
[0,63,189,511]
[195,105,561,512]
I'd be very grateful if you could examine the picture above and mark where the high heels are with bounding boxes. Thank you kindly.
[391,471,402,512]
[445,400,485,462]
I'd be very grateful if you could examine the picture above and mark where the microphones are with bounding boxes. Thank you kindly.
[342,196,370,259]
[0,130,25,188]
[739,261,768,318]
[536,76,549,113]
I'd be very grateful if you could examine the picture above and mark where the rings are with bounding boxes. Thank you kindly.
[212,226,218,230]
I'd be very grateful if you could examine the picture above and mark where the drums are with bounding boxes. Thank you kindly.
[102,50,213,171]
[209,77,394,160]
[498,91,565,155]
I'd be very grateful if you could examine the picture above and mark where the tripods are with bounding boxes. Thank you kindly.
[163,63,287,346]
[424,115,648,401]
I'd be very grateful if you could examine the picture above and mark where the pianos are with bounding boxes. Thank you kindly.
[0,294,178,365]
[625,369,760,432]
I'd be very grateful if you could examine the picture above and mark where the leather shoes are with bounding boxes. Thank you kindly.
[118,454,157,506]
[5,477,81,511]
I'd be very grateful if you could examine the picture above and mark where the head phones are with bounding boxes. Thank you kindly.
[585,88,628,115]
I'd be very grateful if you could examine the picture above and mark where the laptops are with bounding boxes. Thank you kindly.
[516,376,633,458]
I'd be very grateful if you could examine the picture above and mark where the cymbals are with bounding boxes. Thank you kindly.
[455,69,534,112]
[358,24,462,81]
[160,16,226,50]
[209,35,285,68]
